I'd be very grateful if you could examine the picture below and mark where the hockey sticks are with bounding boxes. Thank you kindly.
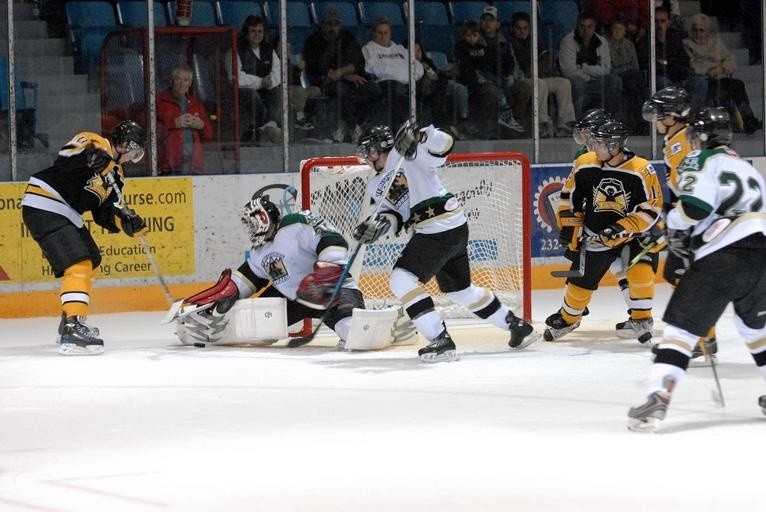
[289,155,405,349]
[621,236,665,274]
[107,173,198,317]
[551,241,586,278]
[683,257,725,406]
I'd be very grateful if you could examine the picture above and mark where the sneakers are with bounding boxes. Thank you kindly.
[743,117,763,134]
[258,121,282,142]
[294,114,313,130]
[498,113,525,132]
[334,120,363,144]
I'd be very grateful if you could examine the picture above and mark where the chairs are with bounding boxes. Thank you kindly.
[0,0,739,148]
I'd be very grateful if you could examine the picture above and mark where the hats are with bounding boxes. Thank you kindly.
[321,8,342,24]
[480,6,499,19]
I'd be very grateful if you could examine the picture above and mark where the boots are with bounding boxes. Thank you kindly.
[58,311,103,346]
[629,389,669,418]
[508,309,582,347]
[419,330,456,354]
[615,317,717,358]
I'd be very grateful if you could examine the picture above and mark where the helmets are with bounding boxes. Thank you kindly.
[242,196,280,248]
[572,108,628,153]
[113,120,146,164]
[642,86,732,151]
[357,125,394,164]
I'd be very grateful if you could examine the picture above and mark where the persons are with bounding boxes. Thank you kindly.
[640,85,718,359]
[19,118,147,347]
[570,106,657,331]
[354,122,533,356]
[162,183,420,352]
[628,106,766,420]
[227,3,763,145]
[542,117,666,344]
[137,65,213,176]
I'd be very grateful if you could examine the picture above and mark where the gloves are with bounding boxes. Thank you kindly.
[559,212,586,251]
[87,147,115,176]
[590,224,630,248]
[394,120,422,161]
[353,215,390,244]
[121,207,148,237]
